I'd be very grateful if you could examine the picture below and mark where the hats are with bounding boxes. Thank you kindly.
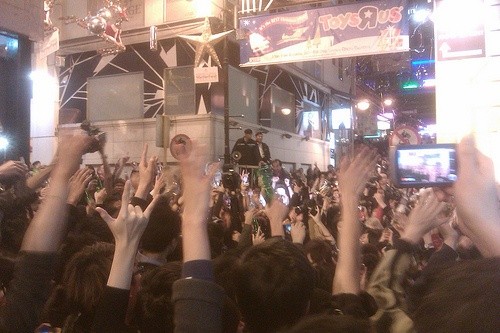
[245,129,252,134]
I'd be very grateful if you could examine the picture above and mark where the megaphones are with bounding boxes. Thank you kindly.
[232,151,241,162]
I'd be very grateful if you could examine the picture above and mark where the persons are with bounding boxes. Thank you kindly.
[0,120,225,333]
[252,130,270,188]
[226,143,500,333]
[230,128,267,185]
[419,157,430,168]
[433,161,445,181]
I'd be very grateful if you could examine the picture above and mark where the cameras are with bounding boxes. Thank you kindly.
[295,199,318,216]
[389,142,458,188]
[284,224,291,234]
[79,120,106,156]
